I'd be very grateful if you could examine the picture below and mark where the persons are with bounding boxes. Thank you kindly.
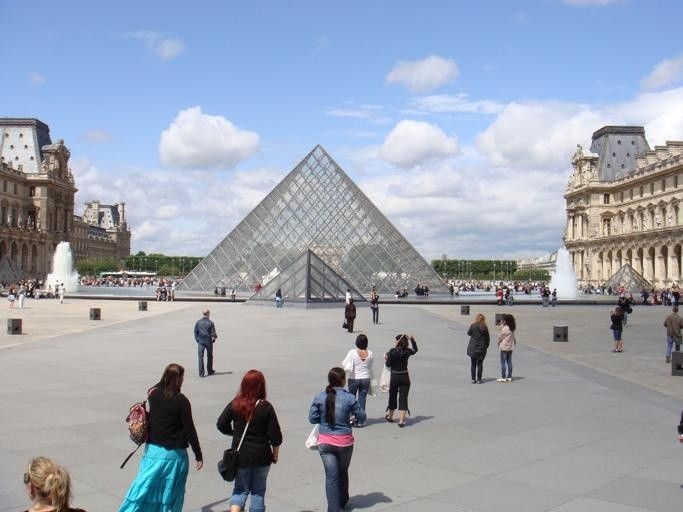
[78,273,176,301]
[678,411,682,443]
[396,288,408,298]
[582,283,635,353]
[254,282,262,293]
[0,277,68,309]
[341,334,374,427]
[117,363,204,512]
[385,334,419,427]
[215,370,282,512]
[214,286,236,301]
[23,457,87,512]
[664,306,682,364]
[496,314,516,382]
[309,368,366,512]
[194,309,217,377]
[466,314,490,384]
[344,289,356,332]
[275,288,282,308]
[449,279,558,307]
[371,286,379,323]
[640,283,683,306]
[415,284,429,296]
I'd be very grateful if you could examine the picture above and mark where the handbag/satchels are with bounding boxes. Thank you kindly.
[217,447,241,481]
[303,423,320,450]
[344,359,354,373]
[343,322,348,329]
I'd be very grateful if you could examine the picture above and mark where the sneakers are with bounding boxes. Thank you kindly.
[496,378,506,382]
[350,415,355,425]
[508,377,512,383]
[357,422,366,426]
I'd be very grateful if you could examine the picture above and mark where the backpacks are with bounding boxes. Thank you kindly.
[125,402,149,447]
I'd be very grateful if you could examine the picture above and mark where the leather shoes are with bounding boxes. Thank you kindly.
[385,415,393,422]
[399,423,404,428]
